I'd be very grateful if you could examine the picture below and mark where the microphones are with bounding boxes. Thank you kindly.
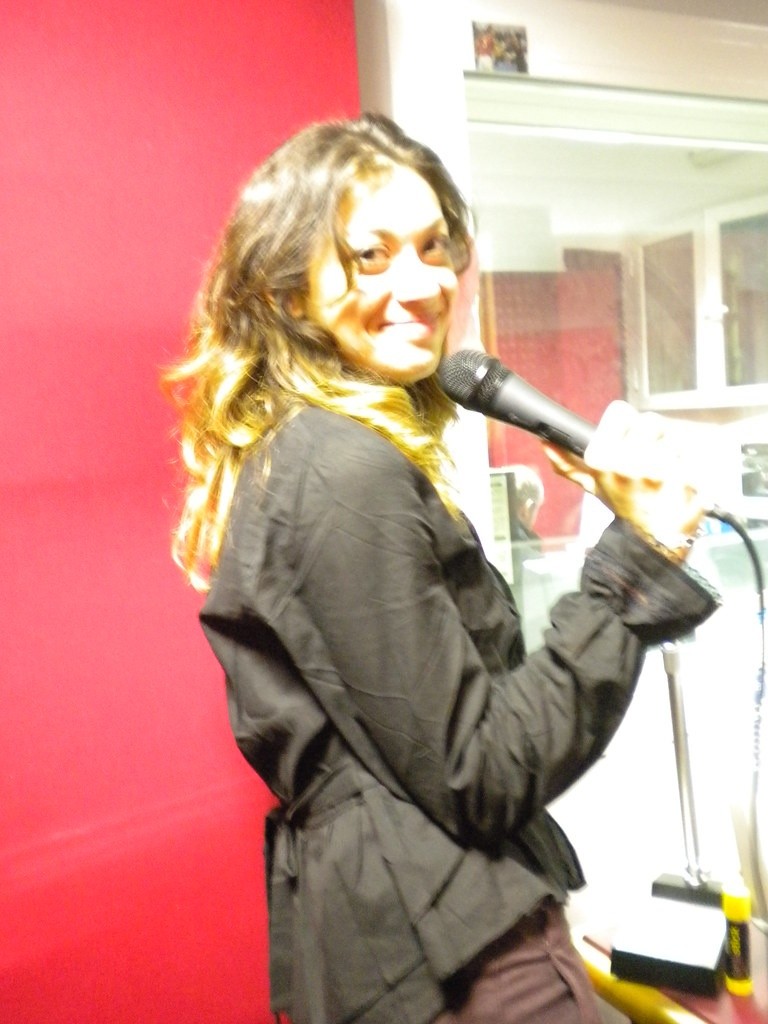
[439,348,729,520]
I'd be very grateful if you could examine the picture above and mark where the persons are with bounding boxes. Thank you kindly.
[489,464,545,585]
[475,24,528,73]
[173,111,709,1024]
